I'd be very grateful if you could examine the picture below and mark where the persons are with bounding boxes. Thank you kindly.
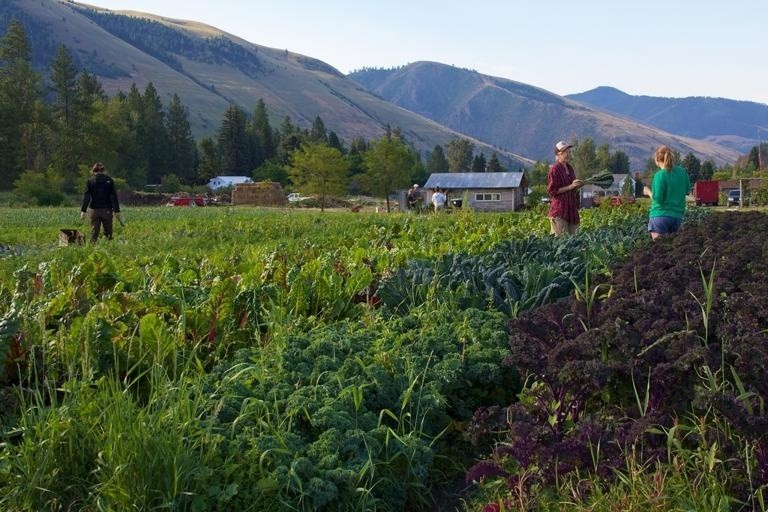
[545,140,586,238]
[430,185,448,214]
[80,161,122,247]
[405,188,418,215]
[413,183,424,204]
[642,144,691,243]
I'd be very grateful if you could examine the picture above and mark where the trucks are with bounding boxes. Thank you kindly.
[694,180,719,207]
[287,193,311,202]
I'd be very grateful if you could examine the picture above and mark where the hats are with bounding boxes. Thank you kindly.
[555,139,574,155]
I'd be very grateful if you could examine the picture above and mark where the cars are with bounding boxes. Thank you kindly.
[727,190,747,206]
